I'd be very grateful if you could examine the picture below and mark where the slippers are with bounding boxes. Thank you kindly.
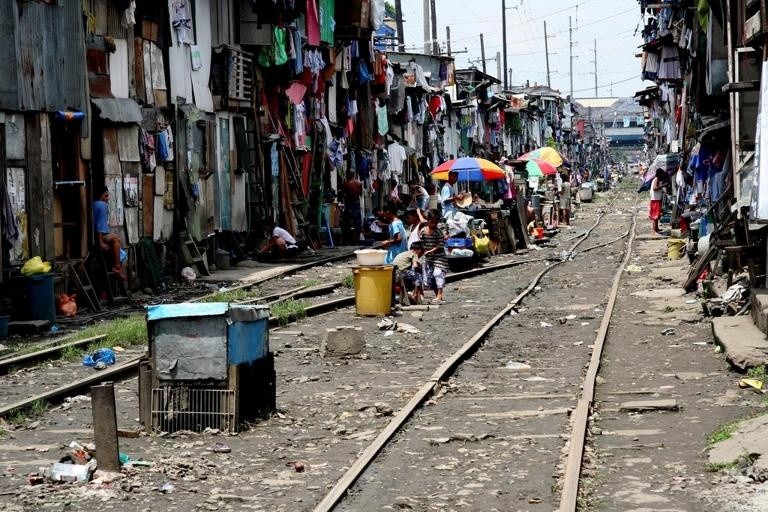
[395,291,446,305]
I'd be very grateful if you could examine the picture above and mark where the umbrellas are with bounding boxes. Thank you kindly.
[426,153,504,195]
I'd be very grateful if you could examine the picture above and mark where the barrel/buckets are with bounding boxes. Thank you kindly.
[353,265,394,316]
[8,272,55,324]
[668,238,685,260]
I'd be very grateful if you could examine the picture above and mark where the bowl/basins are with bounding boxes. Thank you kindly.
[355,248,388,266]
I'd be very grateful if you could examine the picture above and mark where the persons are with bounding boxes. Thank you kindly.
[477,143,623,233]
[408,179,430,210]
[370,204,409,265]
[402,206,428,251]
[256,216,300,260]
[646,167,669,233]
[439,170,482,238]
[371,207,392,236]
[390,241,425,304]
[93,185,130,282]
[418,208,449,303]
